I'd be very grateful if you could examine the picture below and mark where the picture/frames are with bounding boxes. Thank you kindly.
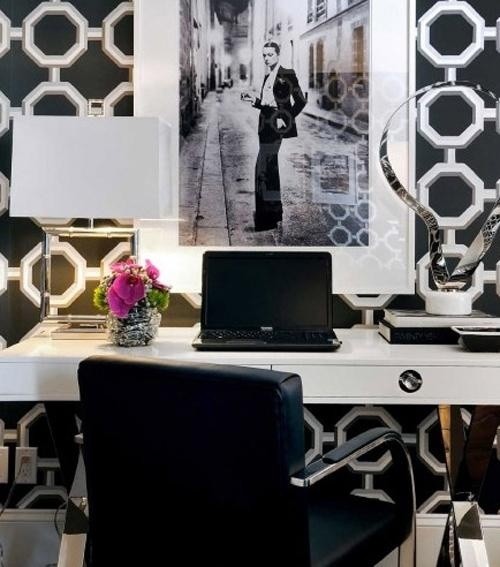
[133,0,416,294]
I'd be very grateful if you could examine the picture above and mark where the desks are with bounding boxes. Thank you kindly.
[2,326,499,406]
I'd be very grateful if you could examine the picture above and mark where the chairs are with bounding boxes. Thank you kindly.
[71,355,415,566]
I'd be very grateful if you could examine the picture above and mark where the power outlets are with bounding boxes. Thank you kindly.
[15,447,38,484]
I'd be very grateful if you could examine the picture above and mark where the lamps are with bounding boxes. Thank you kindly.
[9,116,169,340]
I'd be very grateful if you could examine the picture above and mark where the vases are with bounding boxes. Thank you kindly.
[106,306,161,346]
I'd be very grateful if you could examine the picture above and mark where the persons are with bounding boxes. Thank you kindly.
[241,41,307,231]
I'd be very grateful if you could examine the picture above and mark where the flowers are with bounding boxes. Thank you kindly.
[92,258,170,318]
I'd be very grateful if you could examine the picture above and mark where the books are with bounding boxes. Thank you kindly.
[378,307,496,344]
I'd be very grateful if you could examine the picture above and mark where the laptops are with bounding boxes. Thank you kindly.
[191,251,341,351]
